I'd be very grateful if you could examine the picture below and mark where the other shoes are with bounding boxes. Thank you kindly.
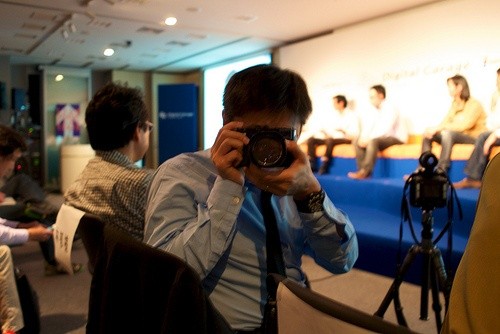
[453,179,483,190]
[348,170,370,181]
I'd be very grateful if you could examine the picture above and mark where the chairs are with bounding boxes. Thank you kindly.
[77,215,221,334]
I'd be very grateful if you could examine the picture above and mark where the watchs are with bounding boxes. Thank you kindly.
[294,186,325,214]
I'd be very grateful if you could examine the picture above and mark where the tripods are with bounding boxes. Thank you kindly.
[374,208,451,334]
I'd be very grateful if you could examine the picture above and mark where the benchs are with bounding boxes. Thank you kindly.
[286,139,500,288]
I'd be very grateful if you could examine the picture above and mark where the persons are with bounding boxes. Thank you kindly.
[144,63,358,334]
[64,82,165,246]
[403,74,486,182]
[308,94,354,175]
[346,85,407,180]
[449,68,500,190]
[0,124,82,334]
[438,149,500,333]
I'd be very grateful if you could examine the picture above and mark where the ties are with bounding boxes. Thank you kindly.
[259,189,288,301]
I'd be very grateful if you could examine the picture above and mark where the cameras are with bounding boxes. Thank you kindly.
[234,125,296,169]
[410,152,449,210]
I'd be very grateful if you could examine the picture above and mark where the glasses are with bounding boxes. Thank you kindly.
[143,120,155,134]
